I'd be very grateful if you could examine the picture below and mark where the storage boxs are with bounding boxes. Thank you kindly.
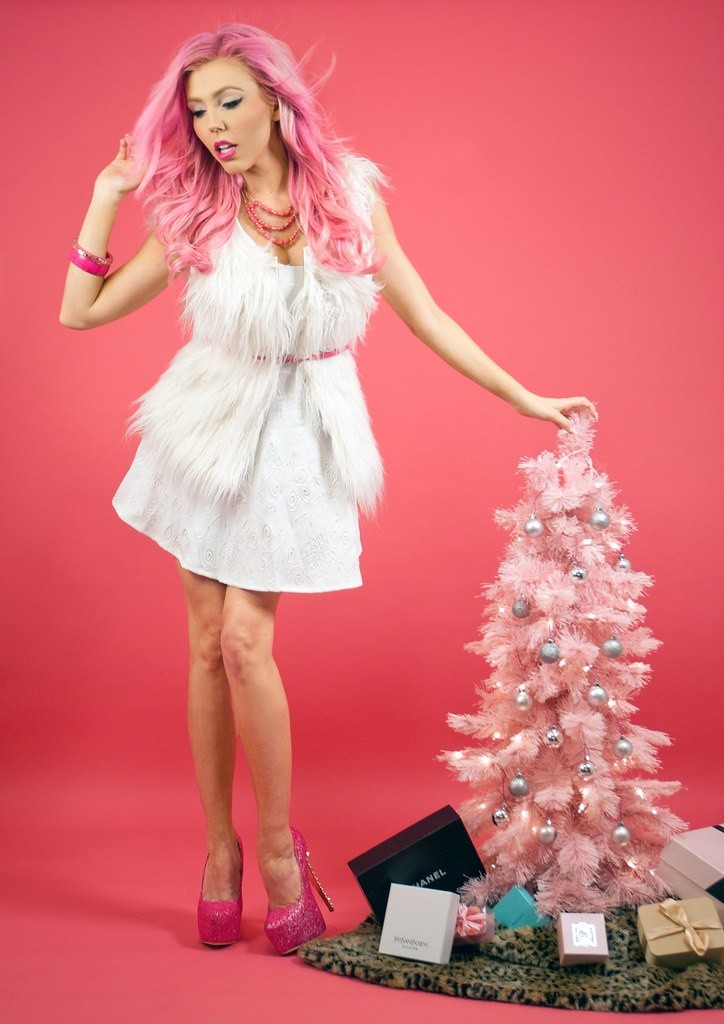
[636,823,724,968]
[492,884,549,930]
[347,806,487,966]
[555,912,609,968]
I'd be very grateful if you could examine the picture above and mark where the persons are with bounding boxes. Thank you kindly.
[59,22,596,956]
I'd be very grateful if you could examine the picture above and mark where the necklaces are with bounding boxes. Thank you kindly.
[239,187,300,245]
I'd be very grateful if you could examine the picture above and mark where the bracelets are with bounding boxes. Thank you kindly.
[70,249,110,276]
[71,239,113,266]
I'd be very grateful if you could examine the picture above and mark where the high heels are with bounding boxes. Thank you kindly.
[264,827,334,955]
[198,830,243,947]
